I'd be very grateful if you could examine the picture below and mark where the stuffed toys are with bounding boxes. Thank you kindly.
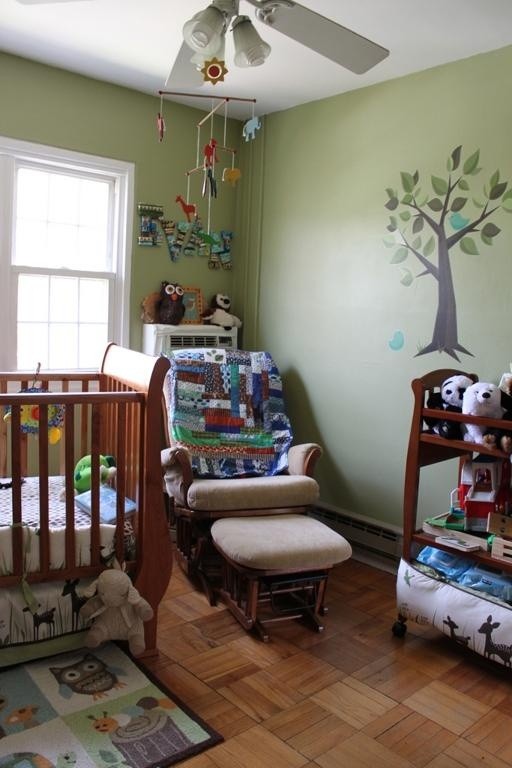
[157,281,186,325]
[60,453,118,501]
[82,569,155,657]
[200,293,243,331]
[426,372,512,453]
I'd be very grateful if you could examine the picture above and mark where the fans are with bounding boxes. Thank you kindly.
[19,0,389,90]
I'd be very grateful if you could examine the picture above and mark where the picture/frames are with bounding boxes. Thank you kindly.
[181,286,204,326]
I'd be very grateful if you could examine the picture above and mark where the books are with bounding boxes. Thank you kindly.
[75,483,136,523]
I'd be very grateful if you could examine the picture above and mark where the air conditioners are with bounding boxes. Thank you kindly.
[141,322,239,357]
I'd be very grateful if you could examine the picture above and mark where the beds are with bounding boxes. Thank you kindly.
[2,340,171,671]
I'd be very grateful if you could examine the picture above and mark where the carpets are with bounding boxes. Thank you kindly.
[0,641,224,768]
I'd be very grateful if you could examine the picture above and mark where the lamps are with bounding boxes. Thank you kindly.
[182,0,273,70]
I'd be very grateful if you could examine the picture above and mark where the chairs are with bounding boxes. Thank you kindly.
[161,345,352,644]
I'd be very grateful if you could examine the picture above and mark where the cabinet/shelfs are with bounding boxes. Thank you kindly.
[391,368,511,686]
